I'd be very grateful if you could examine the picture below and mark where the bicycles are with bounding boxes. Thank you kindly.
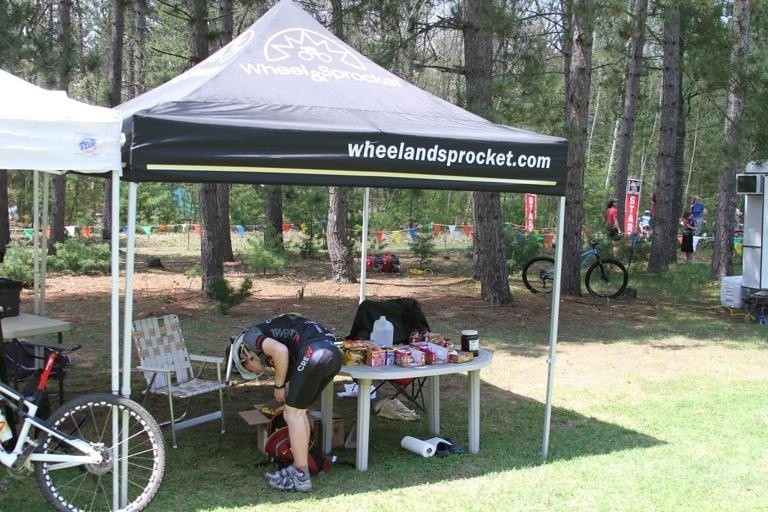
[521,238,629,299]
[0,336,168,512]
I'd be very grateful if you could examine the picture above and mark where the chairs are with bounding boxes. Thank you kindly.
[131,313,227,449]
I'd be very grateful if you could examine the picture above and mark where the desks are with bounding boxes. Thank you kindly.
[316,350,492,473]
[0,311,72,408]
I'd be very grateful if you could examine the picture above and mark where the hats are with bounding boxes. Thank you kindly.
[232,331,265,379]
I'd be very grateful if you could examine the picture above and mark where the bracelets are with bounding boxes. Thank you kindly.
[274,384,285,389]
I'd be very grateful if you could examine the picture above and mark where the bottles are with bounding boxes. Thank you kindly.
[461,329,479,357]
[0,410,14,445]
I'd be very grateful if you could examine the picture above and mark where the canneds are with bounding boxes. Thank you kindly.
[461,329,479,356]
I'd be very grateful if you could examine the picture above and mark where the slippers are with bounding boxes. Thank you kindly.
[438,444,449,456]
[446,437,465,453]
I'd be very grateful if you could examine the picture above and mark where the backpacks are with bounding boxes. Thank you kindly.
[265,410,329,474]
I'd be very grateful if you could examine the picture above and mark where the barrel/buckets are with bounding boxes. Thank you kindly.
[373,316,394,348]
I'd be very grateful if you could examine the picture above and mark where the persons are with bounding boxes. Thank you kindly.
[680,211,696,264]
[690,196,704,235]
[604,198,622,240]
[231,313,342,492]
[641,209,652,232]
[649,191,656,209]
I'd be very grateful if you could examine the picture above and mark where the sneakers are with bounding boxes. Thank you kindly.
[269,476,312,492]
[264,465,294,479]
